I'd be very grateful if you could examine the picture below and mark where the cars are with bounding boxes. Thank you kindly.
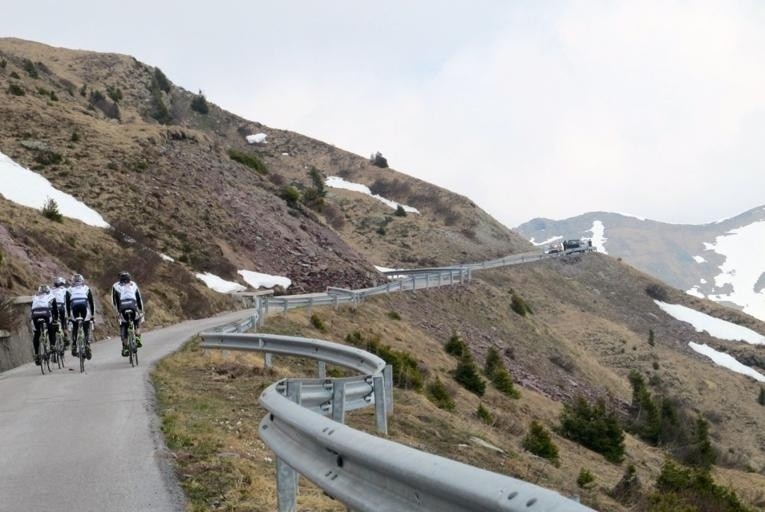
[545,249,560,255]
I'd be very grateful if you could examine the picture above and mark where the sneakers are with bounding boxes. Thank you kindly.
[122,343,129,356]
[72,345,77,356]
[85,345,91,360]
[135,334,142,347]
[35,329,70,365]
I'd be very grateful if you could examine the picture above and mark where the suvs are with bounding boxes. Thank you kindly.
[567,240,585,253]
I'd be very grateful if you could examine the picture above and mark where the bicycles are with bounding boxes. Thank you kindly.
[68,316,95,371]
[114,309,145,367]
[31,319,56,375]
[55,317,72,369]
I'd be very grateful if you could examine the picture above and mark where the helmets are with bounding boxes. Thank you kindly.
[71,274,85,287]
[38,284,50,295]
[118,271,131,284]
[53,277,66,288]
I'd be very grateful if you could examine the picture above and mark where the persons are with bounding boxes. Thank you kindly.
[64,274,97,360]
[110,270,146,358]
[30,284,56,366]
[49,276,70,352]
